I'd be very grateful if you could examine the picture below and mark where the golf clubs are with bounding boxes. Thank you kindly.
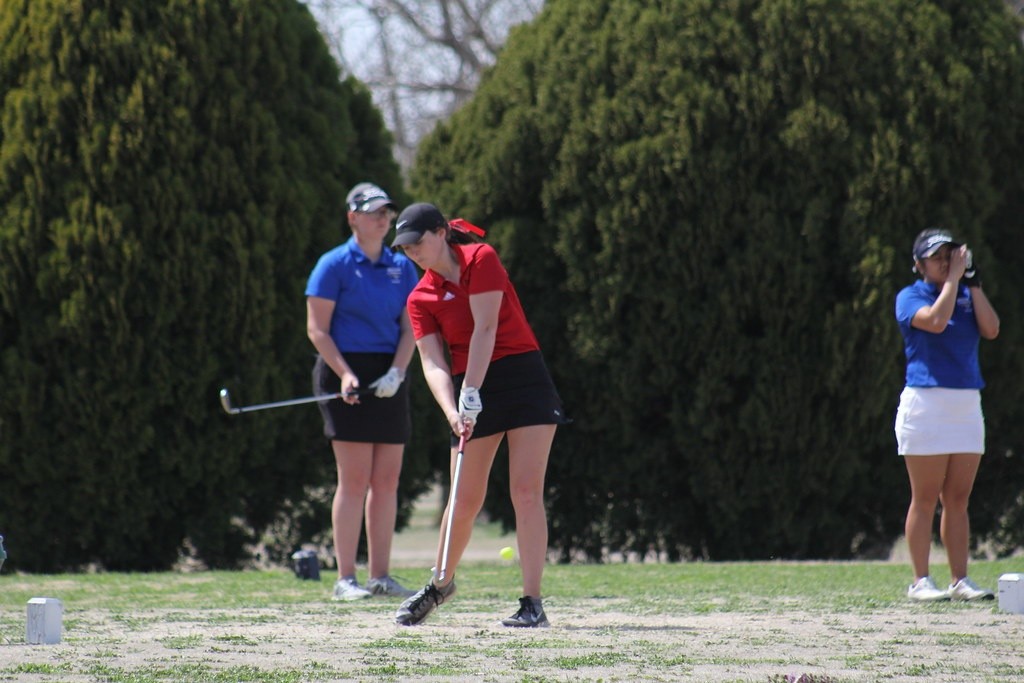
[430,418,469,584]
[219,386,377,417]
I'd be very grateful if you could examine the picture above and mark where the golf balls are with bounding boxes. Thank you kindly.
[500,546,514,560]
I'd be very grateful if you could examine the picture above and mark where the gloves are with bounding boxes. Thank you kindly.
[457,386,481,425]
[369,367,403,398]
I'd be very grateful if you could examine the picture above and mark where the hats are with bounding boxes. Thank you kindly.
[345,182,398,216]
[914,228,959,258]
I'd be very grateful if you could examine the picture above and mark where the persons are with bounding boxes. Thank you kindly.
[388,202,571,630]
[892,227,1000,604]
[304,182,422,603]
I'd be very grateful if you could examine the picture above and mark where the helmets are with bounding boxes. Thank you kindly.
[390,203,450,248]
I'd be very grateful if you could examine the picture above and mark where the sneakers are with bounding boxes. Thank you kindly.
[908,576,950,601]
[333,576,371,602]
[501,597,550,628]
[947,577,994,601]
[395,574,456,625]
[368,576,418,596]
